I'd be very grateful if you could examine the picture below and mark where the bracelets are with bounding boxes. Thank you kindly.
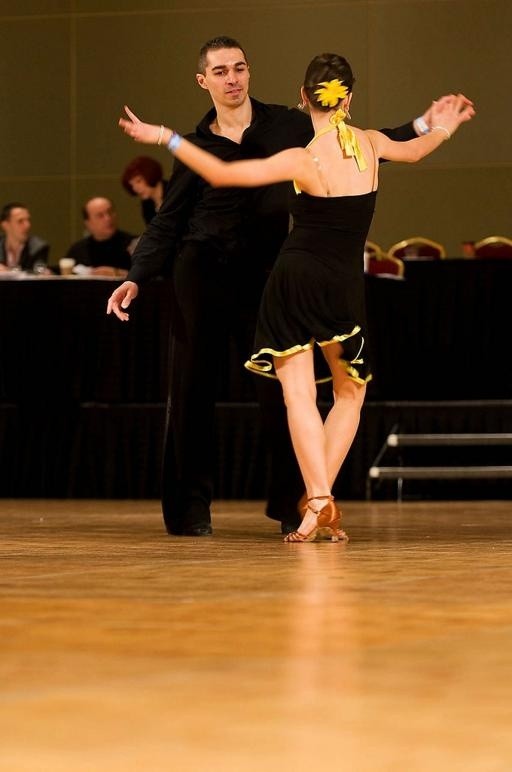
[415,116,430,134]
[432,127,452,140]
[156,124,163,146]
[167,131,181,155]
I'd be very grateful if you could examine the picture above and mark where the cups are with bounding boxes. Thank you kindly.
[460,239,478,256]
[59,258,76,276]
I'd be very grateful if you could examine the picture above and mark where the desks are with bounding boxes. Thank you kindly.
[0,255,512,499]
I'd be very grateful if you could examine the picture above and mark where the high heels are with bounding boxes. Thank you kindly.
[283,491,349,542]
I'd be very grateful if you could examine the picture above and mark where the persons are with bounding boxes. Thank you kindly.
[120,153,170,231]
[0,202,52,278]
[54,197,138,279]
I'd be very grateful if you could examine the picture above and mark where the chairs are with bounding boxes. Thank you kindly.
[355,228,511,283]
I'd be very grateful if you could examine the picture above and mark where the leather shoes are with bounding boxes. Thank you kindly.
[279,521,300,533]
[186,521,213,536]
